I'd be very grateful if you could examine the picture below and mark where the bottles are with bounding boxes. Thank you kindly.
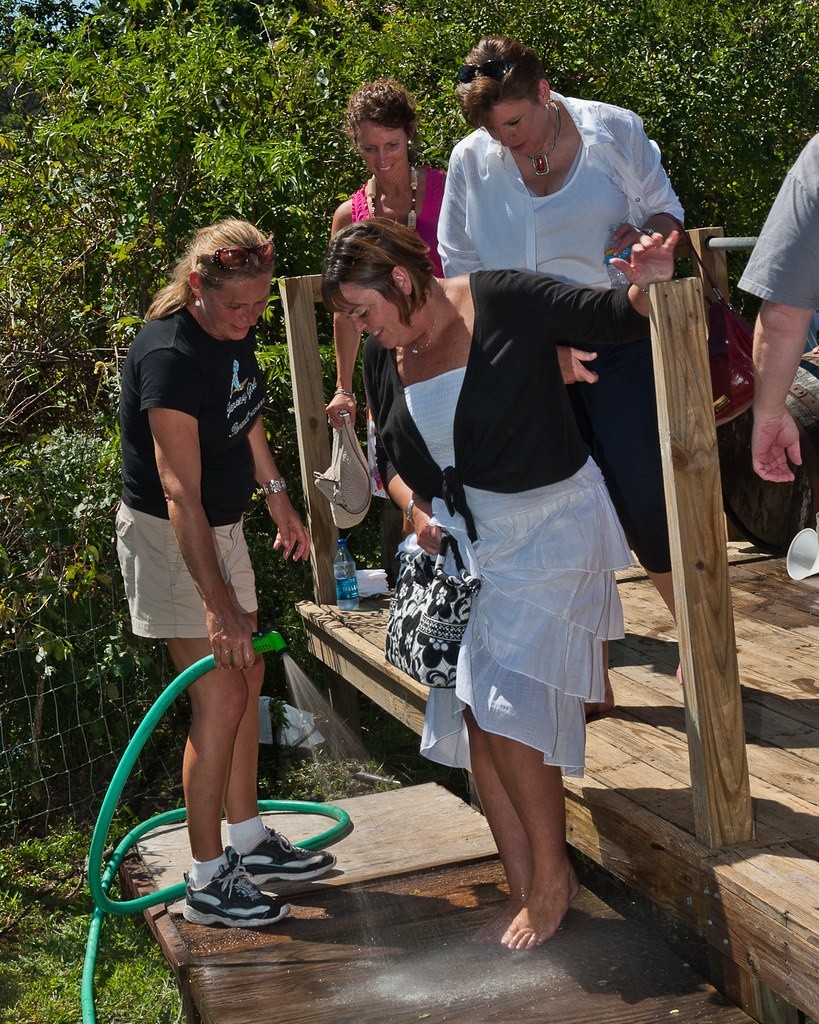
[603,224,632,290]
[333,538,360,611]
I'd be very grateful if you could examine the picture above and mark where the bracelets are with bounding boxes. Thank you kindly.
[406,494,420,527]
[263,476,287,496]
[334,388,353,396]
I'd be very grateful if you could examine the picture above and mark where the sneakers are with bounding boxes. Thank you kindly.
[184,846,292,928]
[226,825,337,885]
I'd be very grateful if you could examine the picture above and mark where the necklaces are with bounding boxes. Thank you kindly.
[527,100,561,176]
[411,278,440,355]
[364,166,419,230]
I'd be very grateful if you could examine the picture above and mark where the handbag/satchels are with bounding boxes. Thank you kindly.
[312,409,374,530]
[385,532,482,690]
[649,209,756,429]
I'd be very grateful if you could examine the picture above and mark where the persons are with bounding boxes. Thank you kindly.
[324,30,685,950]
[114,218,337,928]
[736,132,819,483]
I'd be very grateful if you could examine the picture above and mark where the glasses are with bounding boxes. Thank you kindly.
[202,241,276,271]
[457,59,515,84]
